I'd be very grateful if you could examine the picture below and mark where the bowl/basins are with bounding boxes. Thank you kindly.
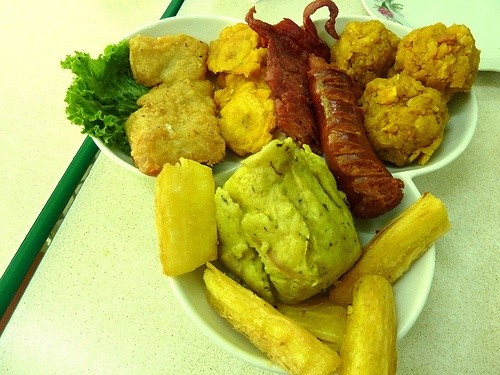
[88,15,478,374]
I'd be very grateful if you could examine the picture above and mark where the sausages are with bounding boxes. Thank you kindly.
[308,51,405,218]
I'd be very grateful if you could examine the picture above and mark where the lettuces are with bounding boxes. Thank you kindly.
[60,38,151,157]
